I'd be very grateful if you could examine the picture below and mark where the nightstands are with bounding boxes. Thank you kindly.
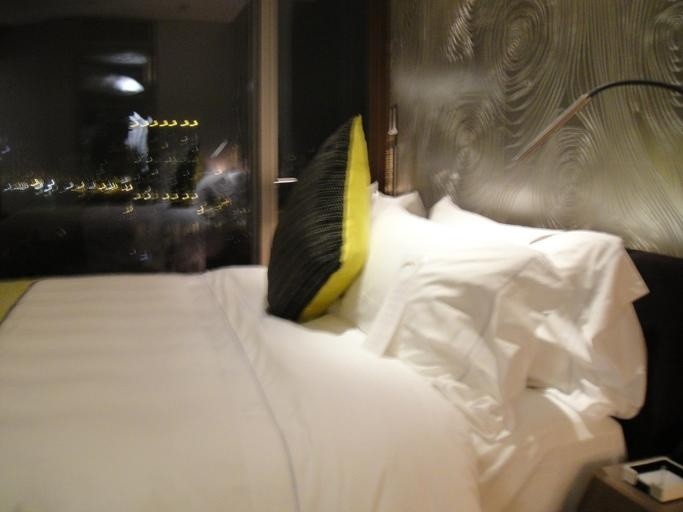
[583,459,682,512]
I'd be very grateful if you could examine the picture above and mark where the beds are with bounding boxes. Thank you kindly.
[0,225,683,512]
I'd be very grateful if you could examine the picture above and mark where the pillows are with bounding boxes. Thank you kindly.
[387,187,428,218]
[265,112,373,320]
[337,189,552,443]
[425,195,649,424]
